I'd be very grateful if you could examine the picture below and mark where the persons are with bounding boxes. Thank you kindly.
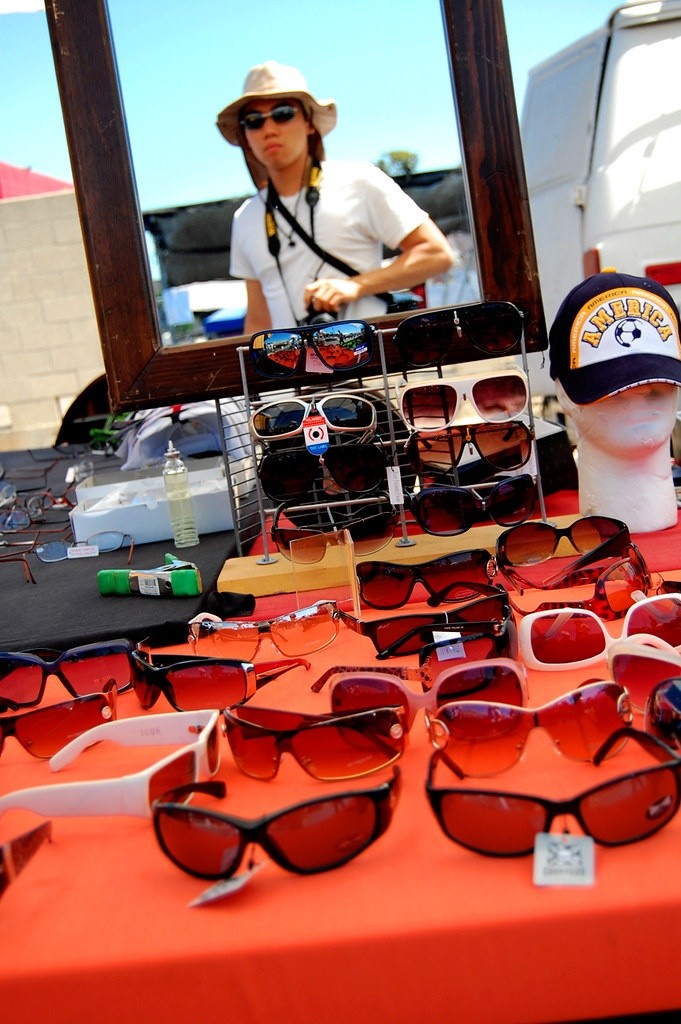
[225,77,456,341]
[554,269,681,534]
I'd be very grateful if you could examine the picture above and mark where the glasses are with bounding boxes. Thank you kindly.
[254,444,388,501]
[400,372,527,431]
[408,423,532,478]
[249,321,374,376]
[247,394,379,444]
[405,487,536,537]
[392,301,523,366]
[0,444,137,586]
[270,500,398,565]
[240,106,304,129]
[0,515,681,881]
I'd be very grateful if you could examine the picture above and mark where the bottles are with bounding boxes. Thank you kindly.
[162,441,200,549]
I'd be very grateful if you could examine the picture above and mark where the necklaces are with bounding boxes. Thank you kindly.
[257,155,306,246]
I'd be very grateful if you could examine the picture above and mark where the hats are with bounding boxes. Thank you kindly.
[546,268,681,406]
[215,61,338,146]
